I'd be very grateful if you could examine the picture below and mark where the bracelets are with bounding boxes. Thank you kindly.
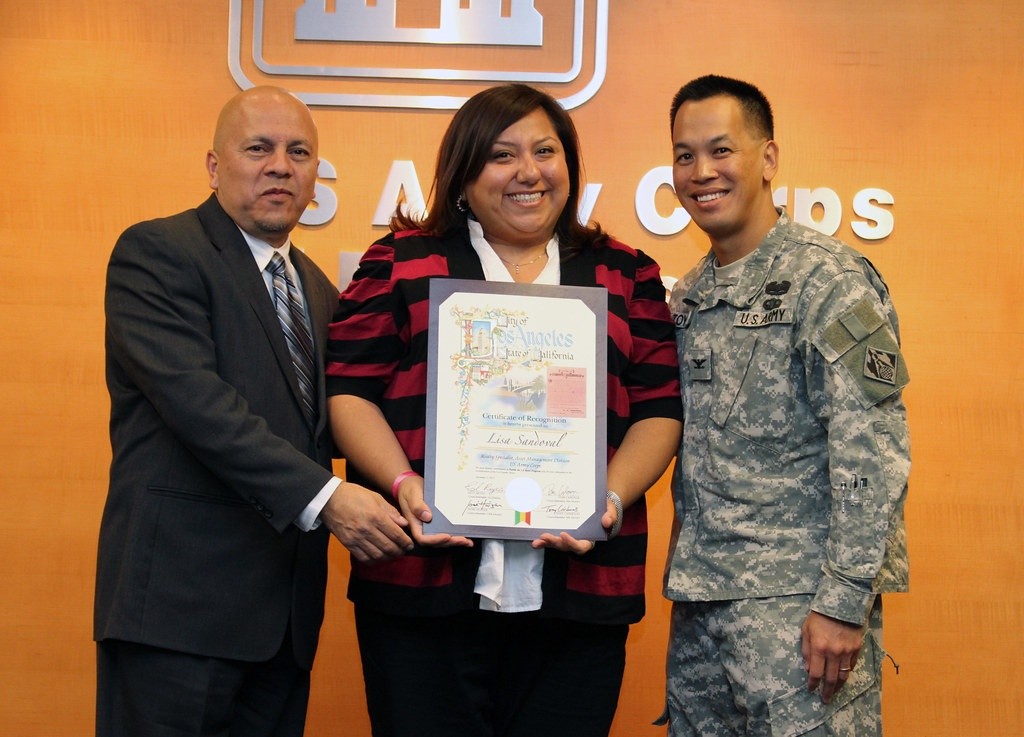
[606,490,622,537]
[392,471,418,500]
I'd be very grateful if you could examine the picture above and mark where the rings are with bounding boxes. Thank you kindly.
[840,668,851,670]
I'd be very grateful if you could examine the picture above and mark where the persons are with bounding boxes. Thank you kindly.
[651,75,914,737]
[324,84,680,737]
[93,84,413,737]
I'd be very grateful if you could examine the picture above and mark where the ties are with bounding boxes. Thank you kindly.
[267,252,316,430]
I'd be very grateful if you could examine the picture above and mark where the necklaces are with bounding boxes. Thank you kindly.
[499,253,545,274]
